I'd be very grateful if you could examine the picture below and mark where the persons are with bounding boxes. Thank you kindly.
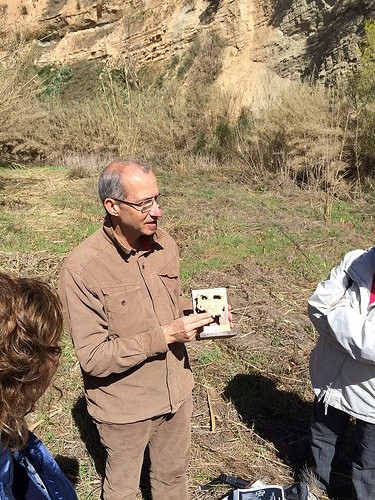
[59,159,234,500]
[298,246,375,500]
[0,270,80,500]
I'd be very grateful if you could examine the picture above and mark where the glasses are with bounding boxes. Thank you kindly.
[113,193,165,213]
